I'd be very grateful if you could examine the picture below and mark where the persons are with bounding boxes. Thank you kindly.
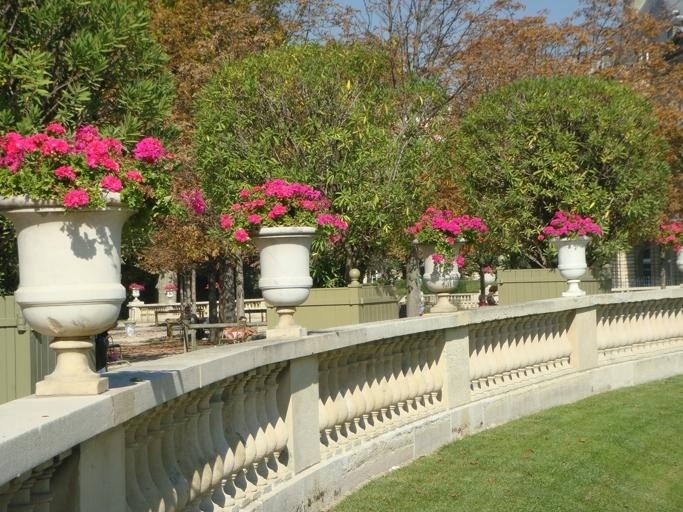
[486,284,497,305]
[477,293,488,306]
[222,316,259,347]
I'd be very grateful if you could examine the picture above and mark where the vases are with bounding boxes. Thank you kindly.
[248,226,317,341]
[130,289,140,302]
[675,247,683,287]
[0,192,139,395]
[164,290,176,304]
[410,236,474,313]
[480,273,499,288]
[547,236,594,299]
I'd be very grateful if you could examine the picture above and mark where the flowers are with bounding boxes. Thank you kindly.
[163,283,177,292]
[656,219,682,252]
[404,207,488,288]
[0,120,166,217]
[537,208,606,247]
[219,180,349,258]
[481,264,497,275]
[127,282,142,290]
